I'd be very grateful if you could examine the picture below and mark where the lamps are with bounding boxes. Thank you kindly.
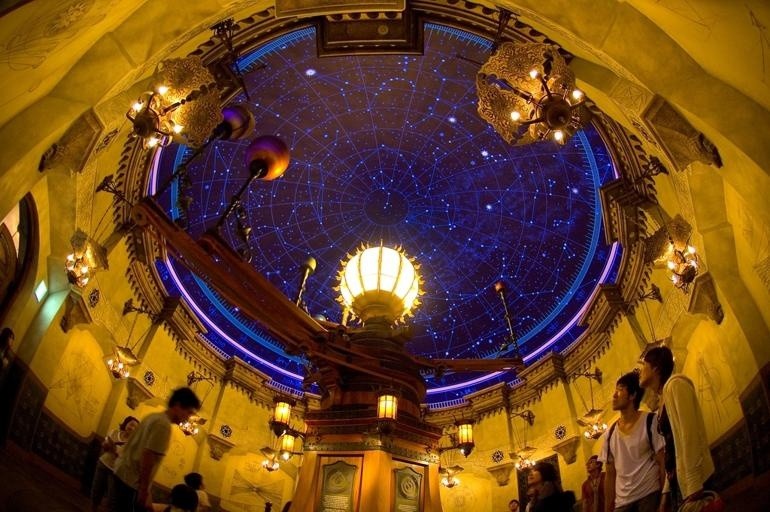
[64,175,133,288]
[126,16,267,151]
[260,380,476,488]
[108,298,161,381]
[456,4,587,147]
[179,370,214,437]
[508,410,538,471]
[624,284,672,364]
[332,239,427,349]
[572,367,607,439]
[633,155,699,294]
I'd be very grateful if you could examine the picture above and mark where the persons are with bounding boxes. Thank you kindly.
[184,472,214,512]
[597,372,668,512]
[0,327,14,370]
[163,484,199,512]
[105,387,200,512]
[509,455,607,512]
[637,345,715,512]
[90,415,140,512]
